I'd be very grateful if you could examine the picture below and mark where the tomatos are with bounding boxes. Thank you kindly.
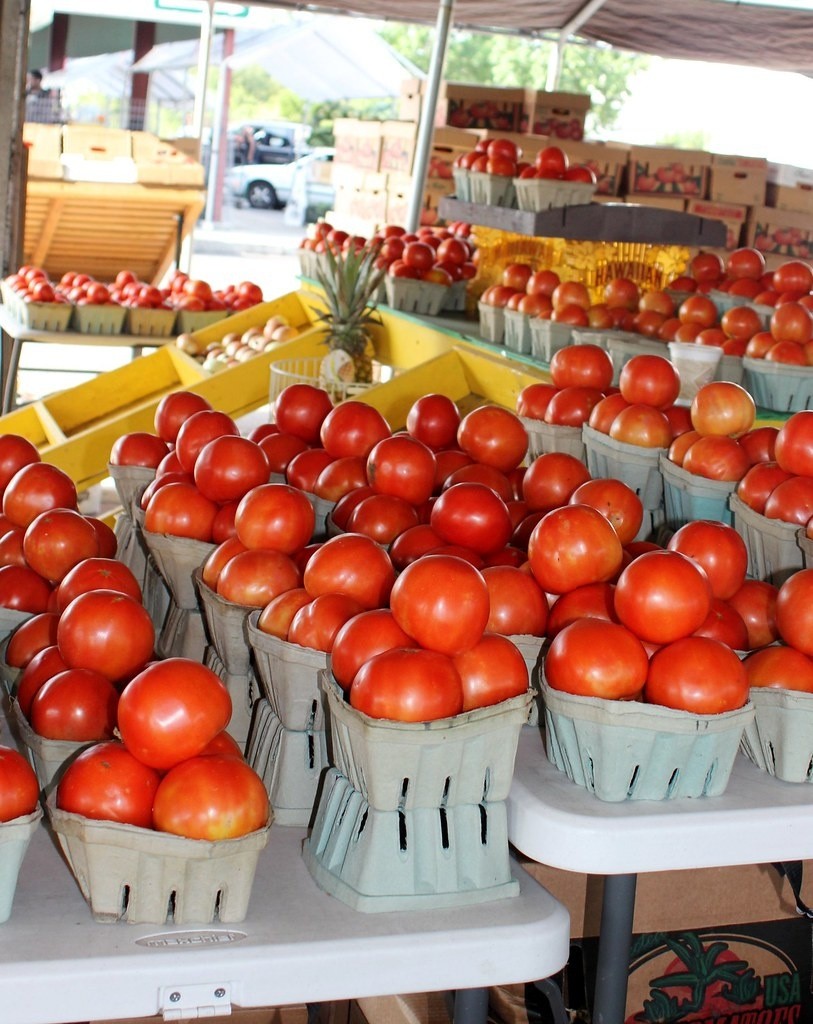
[0,138,813,842]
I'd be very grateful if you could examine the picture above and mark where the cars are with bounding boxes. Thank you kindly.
[226,143,340,210]
[232,121,310,163]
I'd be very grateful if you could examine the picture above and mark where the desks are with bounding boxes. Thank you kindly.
[0,302,178,417]
[0,814,571,1024]
[505,717,812,1024]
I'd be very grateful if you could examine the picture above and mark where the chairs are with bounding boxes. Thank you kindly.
[17,185,205,372]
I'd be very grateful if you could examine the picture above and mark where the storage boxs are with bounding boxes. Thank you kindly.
[0,78,813,1024]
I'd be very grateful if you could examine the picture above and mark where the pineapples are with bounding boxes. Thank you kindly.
[295,232,390,385]
